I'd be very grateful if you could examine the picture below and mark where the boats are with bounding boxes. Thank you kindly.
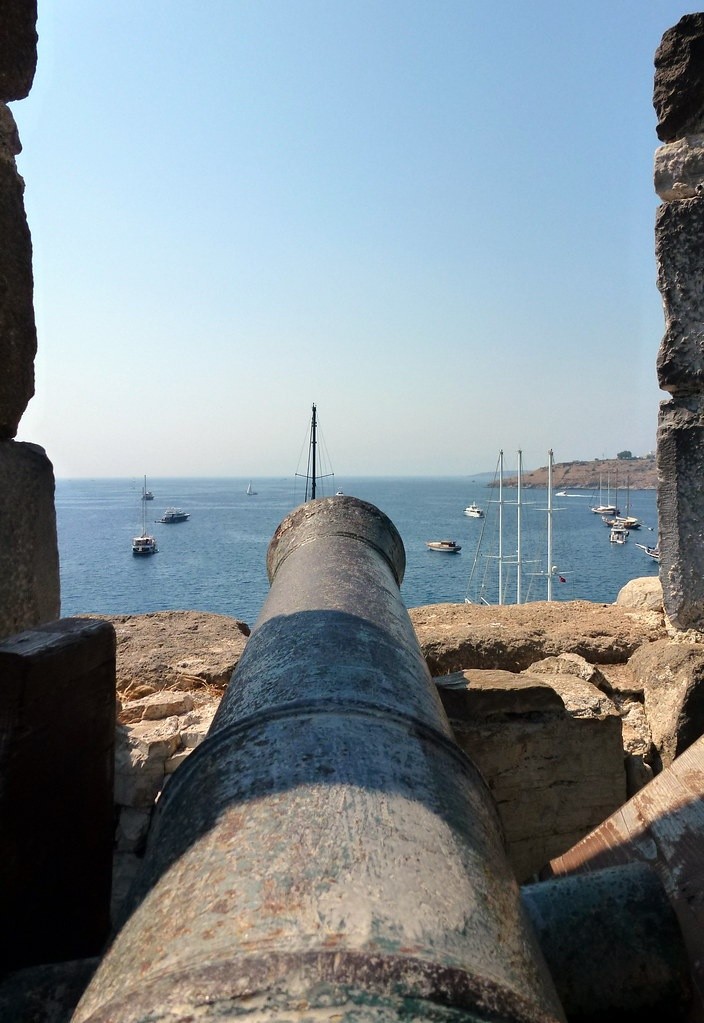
[155,507,191,523]
[607,521,631,545]
[637,542,659,563]
[426,540,462,552]
[335,491,344,496]
[139,491,154,501]
[462,500,485,519]
[132,535,157,554]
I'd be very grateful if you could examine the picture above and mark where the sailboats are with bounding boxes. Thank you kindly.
[246,480,257,495]
[588,471,621,515]
[463,449,577,611]
[602,470,639,528]
